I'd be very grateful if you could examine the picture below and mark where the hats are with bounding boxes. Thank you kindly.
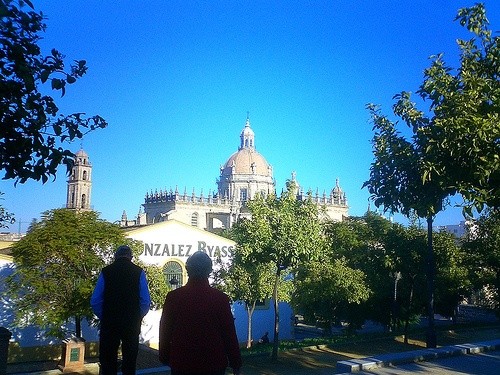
[116,245,131,256]
[186,252,213,274]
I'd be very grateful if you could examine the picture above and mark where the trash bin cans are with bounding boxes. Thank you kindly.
[0,326,12,369]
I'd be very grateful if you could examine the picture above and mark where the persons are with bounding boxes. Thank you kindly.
[90,245,151,375]
[159,250,243,375]
